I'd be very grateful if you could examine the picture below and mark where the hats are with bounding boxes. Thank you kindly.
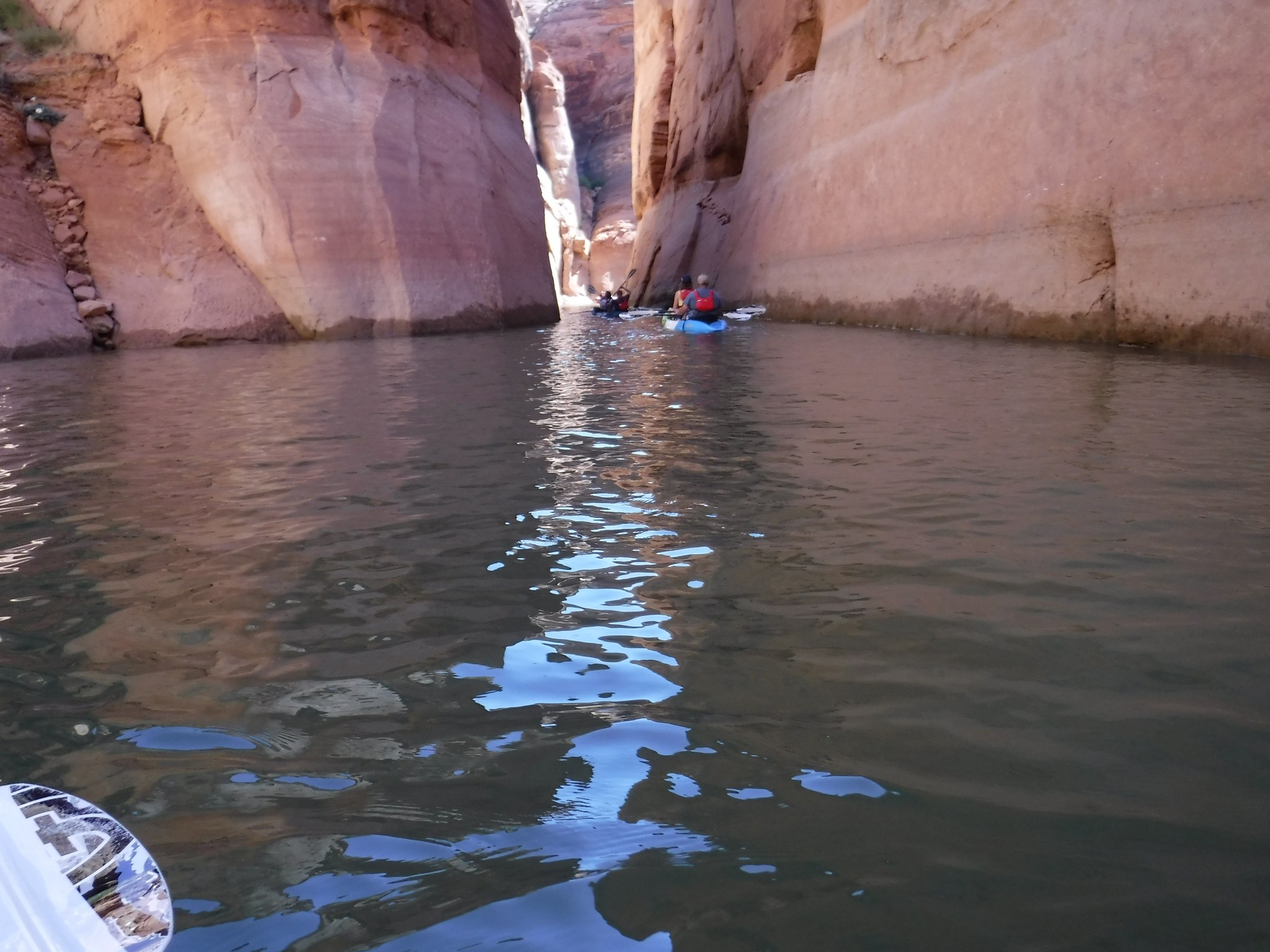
[616,290,623,296]
[605,291,612,297]
[697,274,710,285]
[680,274,691,284]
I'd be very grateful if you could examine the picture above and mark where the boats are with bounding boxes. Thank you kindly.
[661,307,726,335]
[591,305,659,320]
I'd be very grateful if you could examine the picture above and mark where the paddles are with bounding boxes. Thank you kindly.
[618,311,752,321]
[586,283,605,299]
[603,268,637,305]
[627,305,766,319]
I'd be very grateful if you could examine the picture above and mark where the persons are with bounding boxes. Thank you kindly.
[674,274,723,325]
[672,274,694,310]
[611,285,631,311]
[600,290,613,310]
